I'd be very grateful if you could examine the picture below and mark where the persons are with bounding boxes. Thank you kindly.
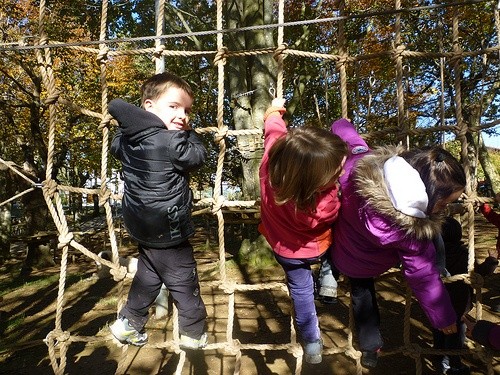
[258,98,347,363]
[432,218,500,375]
[481,193,500,259]
[108,73,208,352]
[465,314,500,354]
[316,117,467,369]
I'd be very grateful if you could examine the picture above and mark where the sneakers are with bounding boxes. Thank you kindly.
[303,337,325,362]
[316,257,341,298]
[180,333,210,350]
[111,316,149,346]
[361,345,382,366]
[443,320,466,350]
